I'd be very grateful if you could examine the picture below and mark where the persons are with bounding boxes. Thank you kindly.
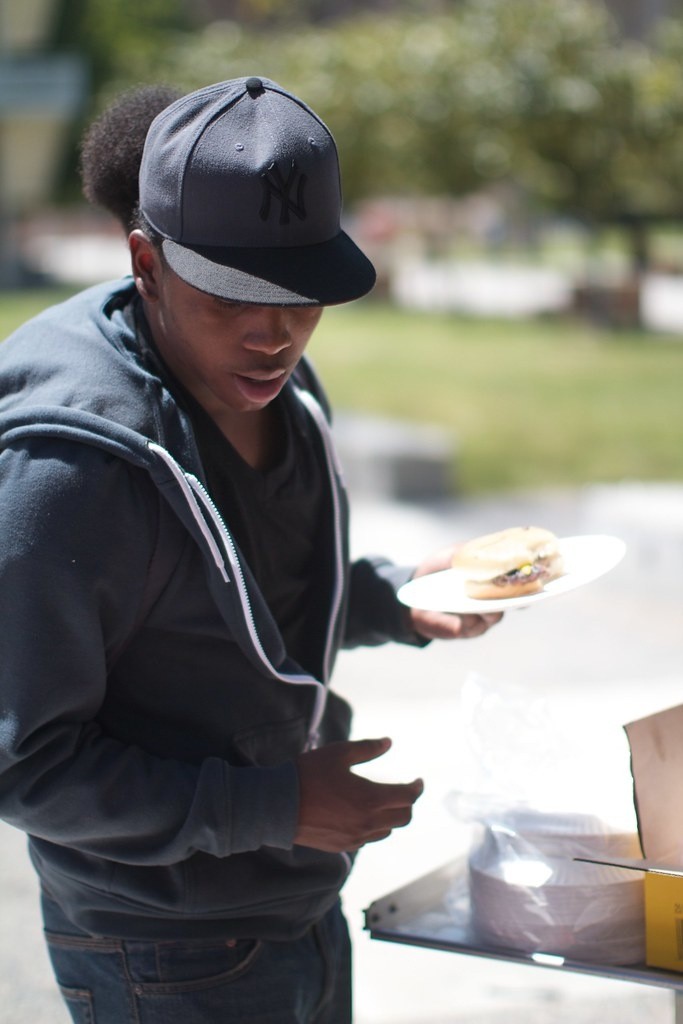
[1,73,507,1024]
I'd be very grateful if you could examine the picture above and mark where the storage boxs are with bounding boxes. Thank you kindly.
[575,855,683,973]
[622,703,683,872]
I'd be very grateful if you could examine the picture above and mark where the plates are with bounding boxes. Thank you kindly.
[398,533,626,616]
[467,835,645,966]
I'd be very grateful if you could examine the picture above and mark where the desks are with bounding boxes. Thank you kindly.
[363,849,683,1024]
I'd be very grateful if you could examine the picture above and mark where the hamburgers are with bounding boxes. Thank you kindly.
[453,526,565,599]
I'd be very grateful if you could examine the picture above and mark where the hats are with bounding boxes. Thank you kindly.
[135,76,375,306]
